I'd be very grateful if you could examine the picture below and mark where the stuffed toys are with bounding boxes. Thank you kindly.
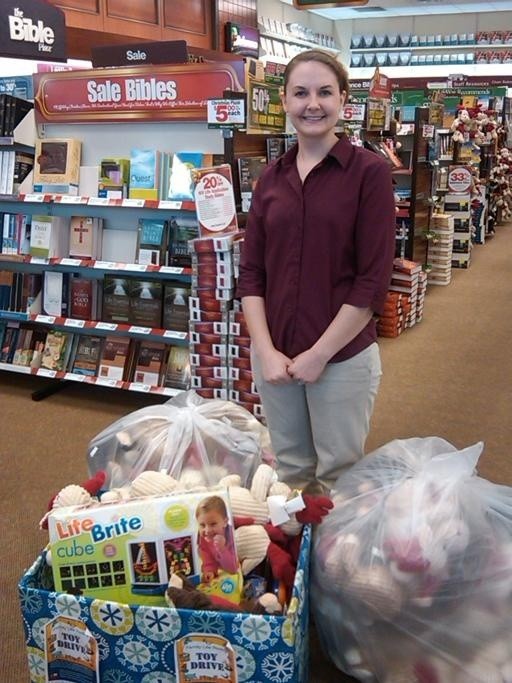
[39,383,512,682]
[40,384,512,683]
[449,105,512,223]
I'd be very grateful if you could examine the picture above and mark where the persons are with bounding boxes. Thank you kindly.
[238,46,397,486]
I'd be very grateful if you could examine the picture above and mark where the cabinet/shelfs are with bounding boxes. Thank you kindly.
[344,70,510,341]
[2,53,296,403]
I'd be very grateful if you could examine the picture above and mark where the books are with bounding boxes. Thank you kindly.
[0,94,298,390]
[341,99,488,335]
[258,13,337,58]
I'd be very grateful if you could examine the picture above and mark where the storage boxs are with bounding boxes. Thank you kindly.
[16,515,312,683]
